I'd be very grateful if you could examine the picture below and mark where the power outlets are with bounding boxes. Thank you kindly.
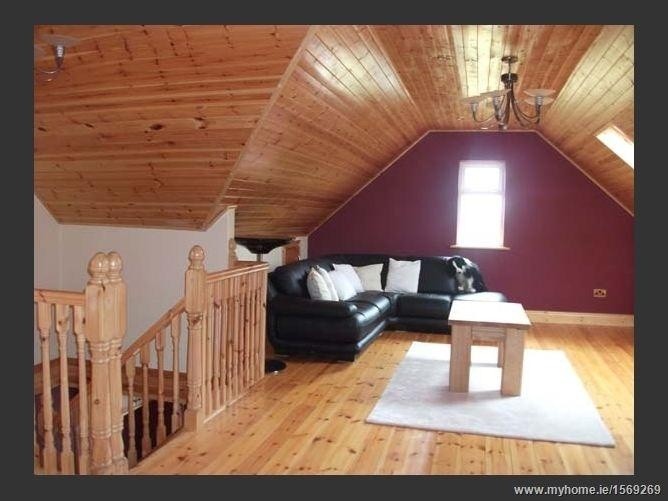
[593,288,607,298]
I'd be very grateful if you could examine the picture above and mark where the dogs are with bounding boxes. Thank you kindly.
[448,257,488,293]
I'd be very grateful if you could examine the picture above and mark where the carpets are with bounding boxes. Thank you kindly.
[363,339,616,450]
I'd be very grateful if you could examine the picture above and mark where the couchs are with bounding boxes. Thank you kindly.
[263,251,509,363]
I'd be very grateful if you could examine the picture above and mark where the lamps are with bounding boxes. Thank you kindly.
[33,33,81,86]
[461,55,557,134]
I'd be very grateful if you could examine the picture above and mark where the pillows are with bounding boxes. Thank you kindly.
[305,256,422,302]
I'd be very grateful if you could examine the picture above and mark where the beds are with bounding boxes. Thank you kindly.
[446,298,532,397]
